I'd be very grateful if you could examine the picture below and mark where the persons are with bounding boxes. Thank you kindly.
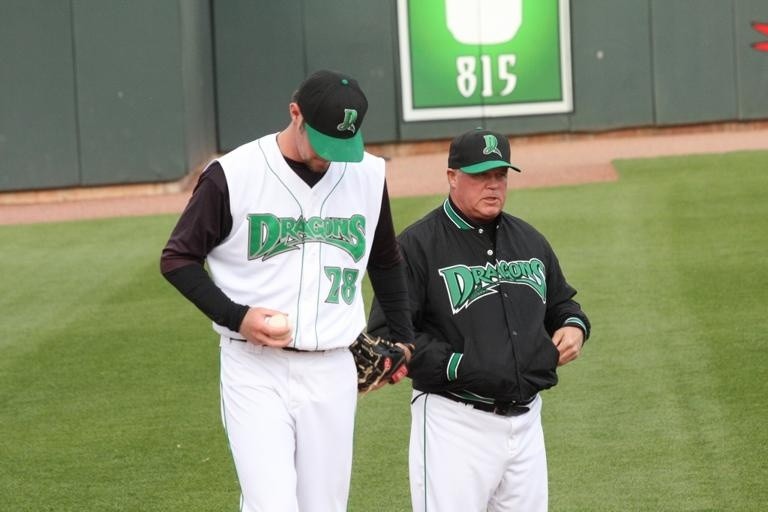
[160,68,416,511]
[367,125,592,512]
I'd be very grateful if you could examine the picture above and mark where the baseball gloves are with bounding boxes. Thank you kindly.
[348,333,415,395]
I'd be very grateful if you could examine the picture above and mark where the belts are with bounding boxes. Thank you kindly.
[435,391,539,416]
[230,335,328,354]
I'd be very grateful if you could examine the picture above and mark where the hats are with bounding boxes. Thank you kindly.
[295,67,370,163]
[446,126,522,176]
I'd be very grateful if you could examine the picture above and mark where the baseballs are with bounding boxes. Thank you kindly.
[265,314,292,341]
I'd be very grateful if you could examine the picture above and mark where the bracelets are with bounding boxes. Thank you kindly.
[401,343,416,355]
